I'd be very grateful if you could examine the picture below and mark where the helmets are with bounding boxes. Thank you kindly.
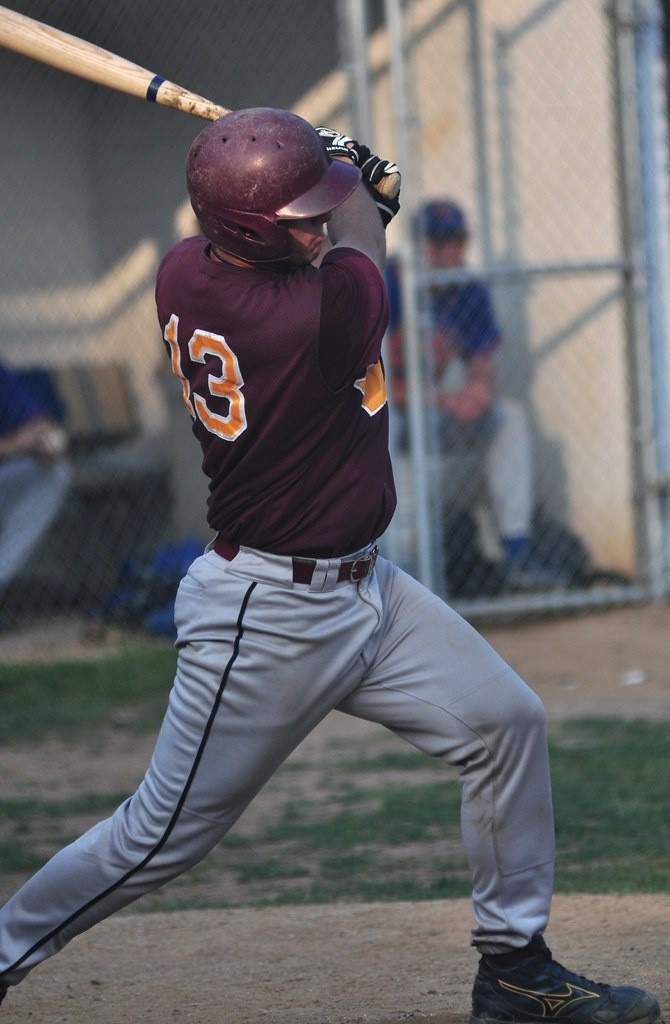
[184,108,359,271]
[411,202,466,238]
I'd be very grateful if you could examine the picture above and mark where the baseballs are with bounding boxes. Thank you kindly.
[36,427,71,458]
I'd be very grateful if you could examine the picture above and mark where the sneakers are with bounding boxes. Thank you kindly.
[472,933,661,1024]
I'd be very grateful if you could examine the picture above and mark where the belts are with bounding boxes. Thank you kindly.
[212,537,379,583]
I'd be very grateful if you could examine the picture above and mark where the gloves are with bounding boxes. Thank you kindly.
[315,125,369,166]
[356,153,403,232]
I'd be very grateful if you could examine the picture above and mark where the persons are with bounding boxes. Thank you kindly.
[0,107,661,1023]
[387,201,565,594]
[0,357,67,594]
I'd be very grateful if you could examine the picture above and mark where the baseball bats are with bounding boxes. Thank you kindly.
[0,3,406,202]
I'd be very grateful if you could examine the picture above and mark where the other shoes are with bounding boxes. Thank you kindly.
[444,554,481,593]
[505,552,570,590]
